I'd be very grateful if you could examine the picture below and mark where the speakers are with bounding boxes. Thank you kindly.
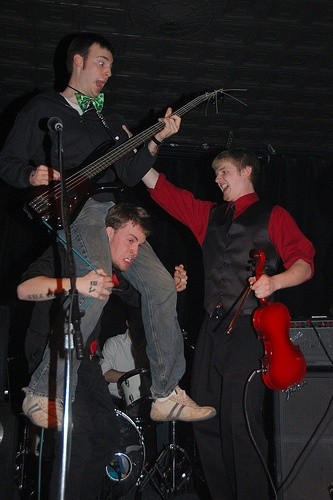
[0,306,21,500]
[261,366,333,500]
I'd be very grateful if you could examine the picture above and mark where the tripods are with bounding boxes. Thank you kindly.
[134,420,207,500]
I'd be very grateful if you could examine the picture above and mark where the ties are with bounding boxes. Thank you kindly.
[225,204,235,233]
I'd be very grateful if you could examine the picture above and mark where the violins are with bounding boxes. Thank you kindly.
[249,247,308,392]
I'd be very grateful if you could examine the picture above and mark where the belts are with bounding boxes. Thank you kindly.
[200,305,254,319]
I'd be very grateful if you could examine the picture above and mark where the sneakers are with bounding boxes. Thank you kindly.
[22,392,74,431]
[150,385,217,421]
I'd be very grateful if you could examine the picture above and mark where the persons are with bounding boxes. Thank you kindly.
[99,319,141,411]
[0,32,216,430]
[115,124,316,500]
[16,203,188,500]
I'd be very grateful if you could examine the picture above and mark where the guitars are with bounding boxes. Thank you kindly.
[0,84,249,258]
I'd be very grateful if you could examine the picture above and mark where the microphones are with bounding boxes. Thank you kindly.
[48,117,63,133]
[109,458,121,479]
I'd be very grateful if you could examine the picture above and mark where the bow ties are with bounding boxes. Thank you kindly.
[76,93,105,113]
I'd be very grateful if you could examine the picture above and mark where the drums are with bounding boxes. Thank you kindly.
[101,408,148,500]
[115,367,153,418]
